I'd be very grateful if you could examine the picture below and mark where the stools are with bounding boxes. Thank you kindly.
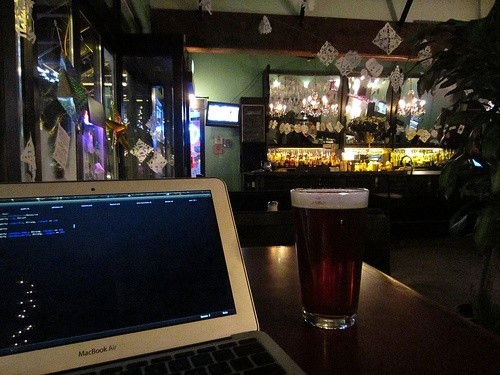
[375,193,403,249]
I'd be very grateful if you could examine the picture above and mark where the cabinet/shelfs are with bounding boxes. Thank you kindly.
[262,63,483,170]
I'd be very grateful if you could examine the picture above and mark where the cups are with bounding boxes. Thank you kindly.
[291,187,368,330]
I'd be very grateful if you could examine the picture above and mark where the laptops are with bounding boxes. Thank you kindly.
[0,177,308,375]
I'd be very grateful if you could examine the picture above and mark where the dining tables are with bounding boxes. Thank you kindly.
[240,247,500,375]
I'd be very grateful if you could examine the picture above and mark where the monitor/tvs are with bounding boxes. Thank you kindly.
[206,102,240,127]
